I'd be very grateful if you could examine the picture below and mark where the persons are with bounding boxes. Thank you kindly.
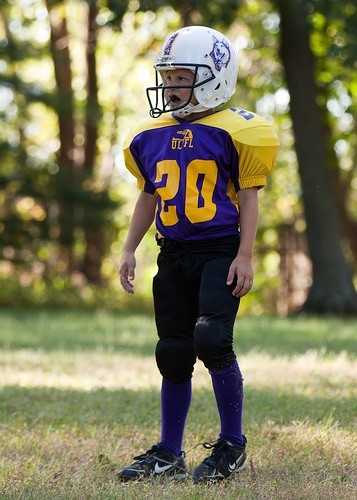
[115,27,281,482]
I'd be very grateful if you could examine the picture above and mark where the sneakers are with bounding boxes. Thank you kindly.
[192,433,248,483]
[119,442,188,483]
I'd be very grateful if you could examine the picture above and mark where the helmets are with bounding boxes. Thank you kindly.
[154,26,240,118]
